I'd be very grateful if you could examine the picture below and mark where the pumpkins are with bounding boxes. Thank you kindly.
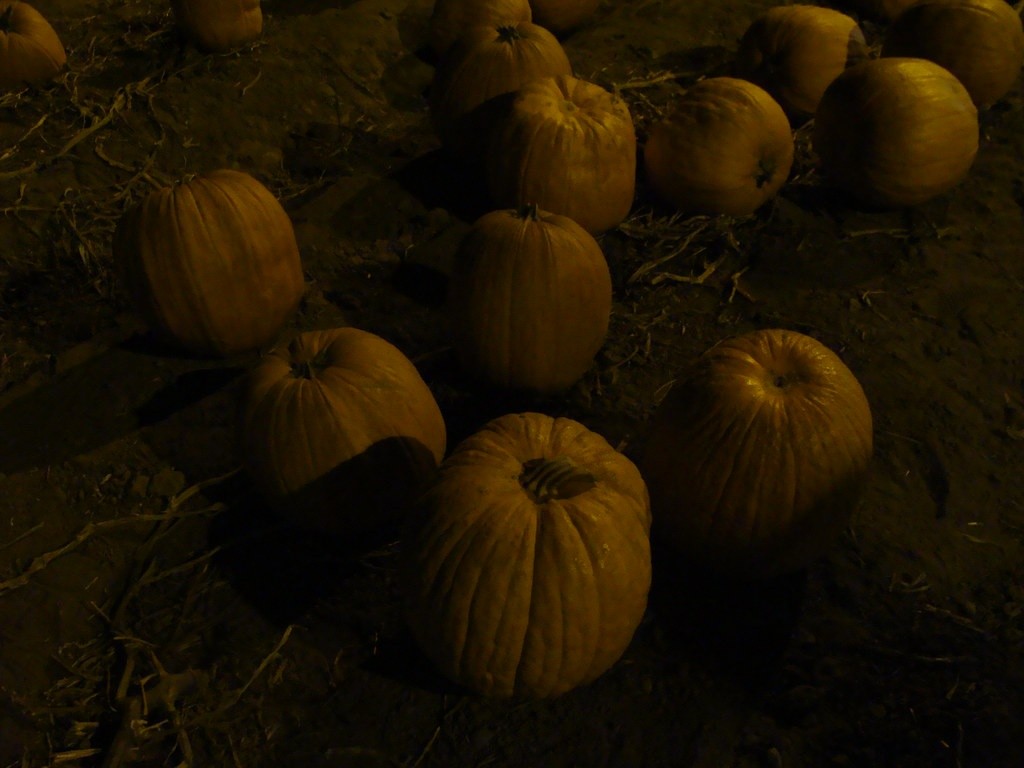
[244,329,448,545]
[642,329,873,580]
[169,0,263,52]
[426,0,1024,235]
[0,0,68,91]
[445,200,614,395]
[403,412,651,701]
[113,168,305,357]
